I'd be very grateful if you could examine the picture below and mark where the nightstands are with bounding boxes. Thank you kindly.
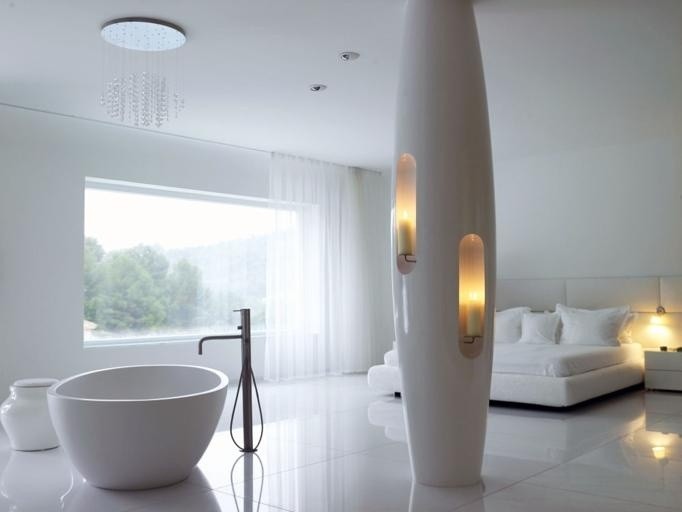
[644,351,682,391]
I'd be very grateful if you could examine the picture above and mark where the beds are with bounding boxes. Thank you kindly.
[367,342,644,407]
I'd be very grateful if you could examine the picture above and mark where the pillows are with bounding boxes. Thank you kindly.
[494,303,629,345]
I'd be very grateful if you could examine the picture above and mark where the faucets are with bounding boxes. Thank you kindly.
[198,335,242,355]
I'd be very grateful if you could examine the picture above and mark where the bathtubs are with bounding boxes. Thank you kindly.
[48,365,228,491]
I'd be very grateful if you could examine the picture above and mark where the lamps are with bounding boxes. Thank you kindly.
[459,235,484,337]
[396,152,416,262]
